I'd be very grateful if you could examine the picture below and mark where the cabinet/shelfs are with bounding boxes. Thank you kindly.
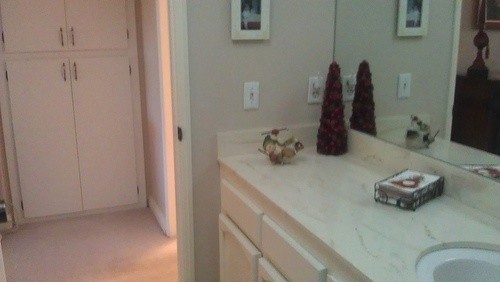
[0,0,148,225]
[216,178,329,282]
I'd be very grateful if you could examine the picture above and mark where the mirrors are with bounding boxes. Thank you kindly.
[334,0,500,181]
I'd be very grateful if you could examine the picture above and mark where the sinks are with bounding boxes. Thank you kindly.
[413,240,500,282]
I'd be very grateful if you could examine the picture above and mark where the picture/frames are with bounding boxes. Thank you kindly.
[476,0,500,30]
[231,0,269,41]
[397,0,428,37]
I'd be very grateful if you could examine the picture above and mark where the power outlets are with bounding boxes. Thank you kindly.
[309,76,322,105]
[343,75,355,98]
